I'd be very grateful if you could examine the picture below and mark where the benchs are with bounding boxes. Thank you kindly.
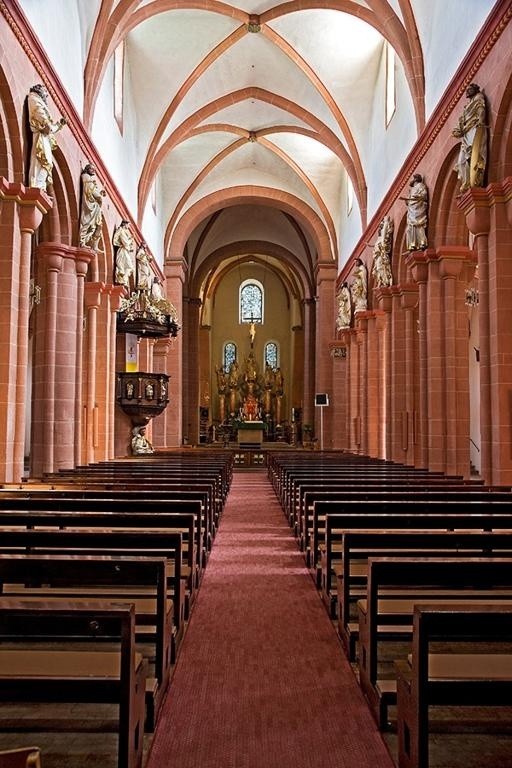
[265,450,510,768]
[2,448,233,767]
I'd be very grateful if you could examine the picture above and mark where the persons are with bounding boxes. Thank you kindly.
[350,258,368,314]
[449,83,488,200]
[79,163,107,255]
[152,276,165,302]
[136,240,154,289]
[27,83,68,191]
[131,425,155,454]
[366,216,394,288]
[113,219,135,285]
[398,174,428,256]
[335,282,352,331]
